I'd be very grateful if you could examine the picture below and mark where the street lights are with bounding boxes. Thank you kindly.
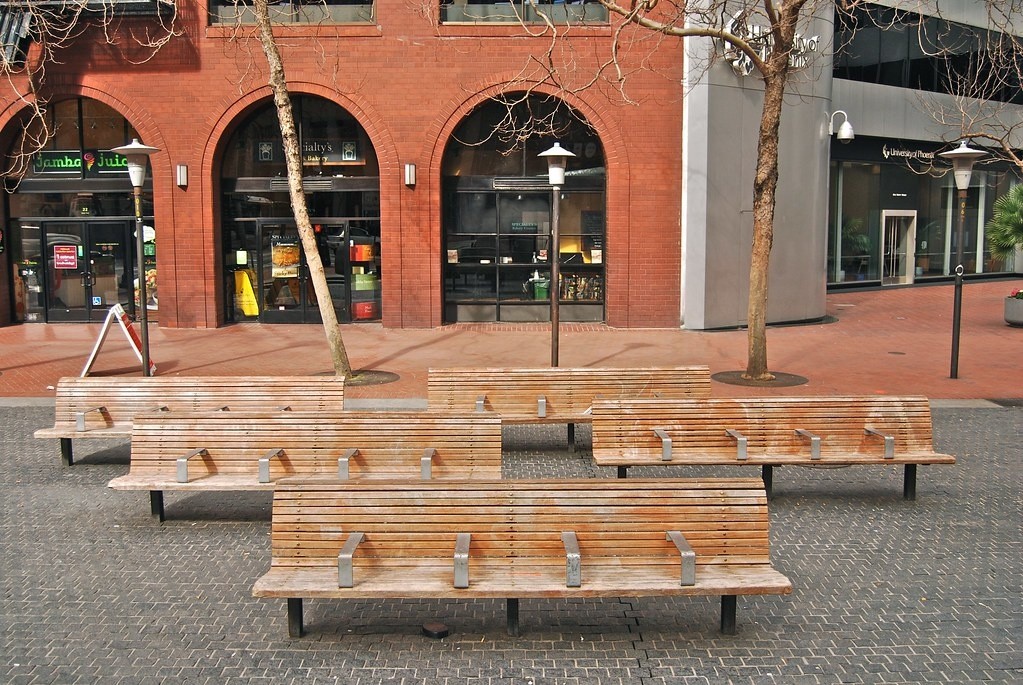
[937,139,989,381]
[535,142,576,367]
[112,137,162,377]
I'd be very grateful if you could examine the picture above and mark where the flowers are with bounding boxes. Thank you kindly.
[1008,287,1023,300]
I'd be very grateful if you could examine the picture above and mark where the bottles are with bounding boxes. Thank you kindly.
[532,252,538,263]
[534,269,539,280]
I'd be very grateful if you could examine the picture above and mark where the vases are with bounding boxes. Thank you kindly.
[1003,296,1023,329]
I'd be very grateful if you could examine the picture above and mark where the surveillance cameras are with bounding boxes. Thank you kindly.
[836,120,855,145]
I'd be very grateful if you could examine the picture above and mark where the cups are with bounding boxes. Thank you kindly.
[500,256,513,264]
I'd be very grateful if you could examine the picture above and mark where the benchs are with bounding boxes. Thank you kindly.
[426,364,714,454]
[590,393,957,504]
[249,476,794,639]
[105,411,503,523]
[32,375,347,466]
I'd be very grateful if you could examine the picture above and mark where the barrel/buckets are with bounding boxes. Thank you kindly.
[528,278,550,299]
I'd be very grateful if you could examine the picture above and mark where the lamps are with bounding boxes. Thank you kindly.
[109,137,160,188]
[940,140,990,191]
[537,141,578,186]
